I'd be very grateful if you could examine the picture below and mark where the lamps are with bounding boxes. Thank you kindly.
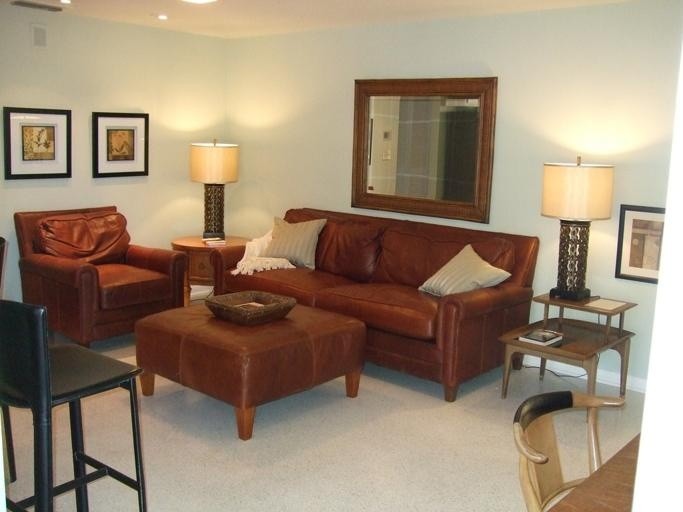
[541,162,614,301]
[190,144,240,238]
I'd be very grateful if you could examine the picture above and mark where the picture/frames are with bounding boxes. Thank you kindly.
[615,204,665,284]
[3,106,72,179]
[92,112,149,178]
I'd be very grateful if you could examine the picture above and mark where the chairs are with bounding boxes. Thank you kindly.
[514,390,625,512]
[0,298,147,511]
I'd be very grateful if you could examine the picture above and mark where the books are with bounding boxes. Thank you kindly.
[203,237,226,247]
[518,328,563,346]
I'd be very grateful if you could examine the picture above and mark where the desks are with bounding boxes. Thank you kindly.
[496,293,638,422]
[546,433,640,511]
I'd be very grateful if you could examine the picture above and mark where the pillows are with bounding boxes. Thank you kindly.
[232,231,296,275]
[259,217,327,271]
[418,243,512,297]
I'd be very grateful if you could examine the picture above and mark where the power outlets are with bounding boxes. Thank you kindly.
[383,150,392,161]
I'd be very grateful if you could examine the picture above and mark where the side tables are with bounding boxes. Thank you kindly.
[172,235,251,307]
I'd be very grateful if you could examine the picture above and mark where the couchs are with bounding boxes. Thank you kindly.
[210,208,540,402]
[14,206,189,347]
[137,303,367,440]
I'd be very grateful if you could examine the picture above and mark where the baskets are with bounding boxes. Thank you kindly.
[205,291,296,325]
